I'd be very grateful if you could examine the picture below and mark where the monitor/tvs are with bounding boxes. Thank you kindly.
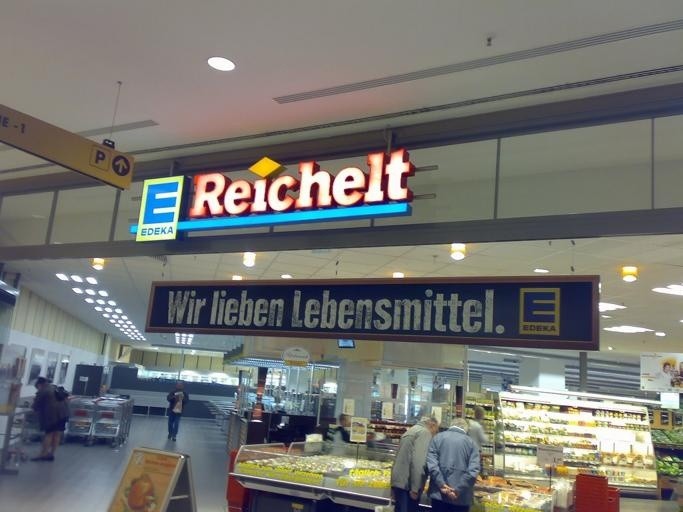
[337,338,355,348]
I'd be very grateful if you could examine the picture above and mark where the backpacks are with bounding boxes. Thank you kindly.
[55,385,69,400]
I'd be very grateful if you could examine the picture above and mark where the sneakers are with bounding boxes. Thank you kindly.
[167,433,177,442]
[30,454,54,461]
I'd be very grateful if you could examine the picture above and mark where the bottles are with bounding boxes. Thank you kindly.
[594,410,648,431]
[465,400,493,469]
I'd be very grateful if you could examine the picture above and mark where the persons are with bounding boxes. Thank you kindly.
[166,380,189,441]
[465,417,490,476]
[31,376,71,461]
[334,414,357,444]
[390,415,441,512]
[426,418,483,512]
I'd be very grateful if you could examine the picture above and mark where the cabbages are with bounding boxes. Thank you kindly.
[655,455,683,476]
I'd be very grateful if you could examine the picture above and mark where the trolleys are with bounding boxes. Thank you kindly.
[1,393,135,463]
